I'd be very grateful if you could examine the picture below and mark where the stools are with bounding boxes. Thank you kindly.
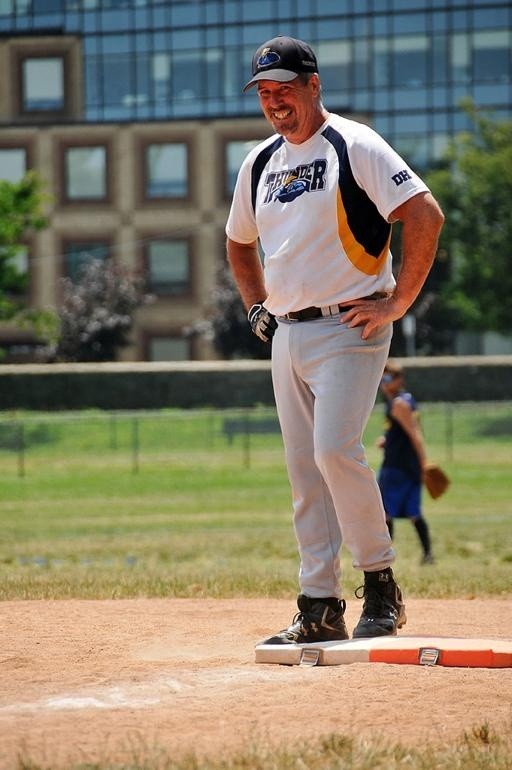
[276,291,390,324]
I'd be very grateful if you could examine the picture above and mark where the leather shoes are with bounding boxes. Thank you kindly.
[246,300,279,344]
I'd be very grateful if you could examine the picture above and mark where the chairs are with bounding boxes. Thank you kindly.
[255,593,350,646]
[352,580,407,638]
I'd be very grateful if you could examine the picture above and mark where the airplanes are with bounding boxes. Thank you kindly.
[425,466,450,499]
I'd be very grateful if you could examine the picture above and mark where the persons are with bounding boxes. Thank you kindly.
[370,359,449,564]
[222,33,450,647]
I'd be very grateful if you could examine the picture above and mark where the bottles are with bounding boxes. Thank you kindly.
[241,34,319,92]
